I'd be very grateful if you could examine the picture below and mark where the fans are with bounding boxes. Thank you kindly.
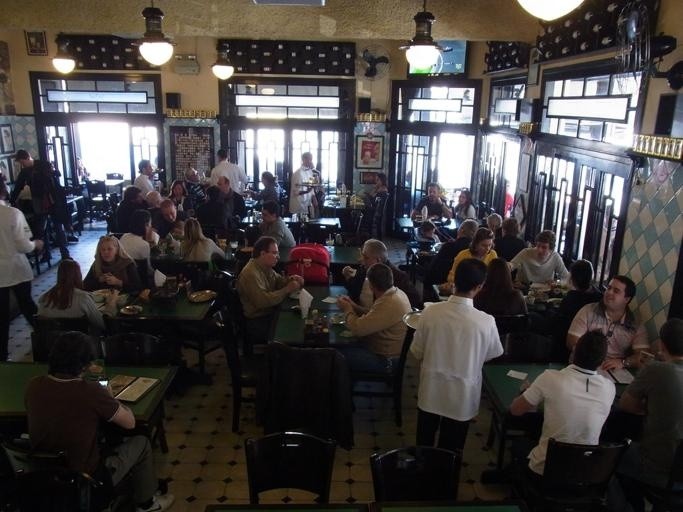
[614,2,682,92]
[355,43,393,84]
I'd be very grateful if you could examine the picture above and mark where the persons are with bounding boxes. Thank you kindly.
[486,211,503,238]
[408,219,441,252]
[565,275,651,373]
[549,259,603,336]
[368,173,389,203]
[8,149,69,259]
[148,199,187,248]
[250,170,279,202]
[115,186,148,234]
[448,227,498,286]
[133,160,156,192]
[287,223,331,285]
[491,329,617,486]
[0,173,46,363]
[494,218,528,261]
[473,258,527,313]
[37,258,109,342]
[337,263,414,412]
[287,151,320,217]
[197,186,237,230]
[454,189,477,219]
[234,236,304,343]
[209,148,250,196]
[313,172,326,209]
[422,219,479,302]
[342,239,424,312]
[120,208,156,278]
[404,258,504,451]
[183,166,209,199]
[509,230,573,287]
[618,317,682,512]
[247,201,296,250]
[146,190,163,212]
[177,216,226,262]
[216,176,248,220]
[168,180,188,212]
[411,183,453,220]
[166,220,185,241]
[25,331,175,512]
[83,235,143,291]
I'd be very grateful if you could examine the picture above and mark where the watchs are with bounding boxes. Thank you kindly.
[621,357,631,368]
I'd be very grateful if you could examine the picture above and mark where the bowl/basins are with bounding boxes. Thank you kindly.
[91,289,112,304]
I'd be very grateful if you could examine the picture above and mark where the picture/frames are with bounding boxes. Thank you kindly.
[9,155,24,184]
[0,156,13,186]
[0,123,16,154]
[355,135,385,170]
[513,194,526,226]
[518,152,532,193]
[359,172,378,185]
[24,28,49,57]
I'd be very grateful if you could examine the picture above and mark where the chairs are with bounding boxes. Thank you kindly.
[244,433,339,505]
[369,443,463,508]
[26,212,52,274]
[107,173,124,200]
[505,330,552,363]
[101,313,164,336]
[0,439,136,512]
[360,193,390,241]
[285,221,302,244]
[135,258,149,287]
[30,317,81,363]
[350,308,420,428]
[523,438,612,512]
[109,333,170,455]
[303,221,339,246]
[5,470,82,512]
[220,310,261,433]
[175,260,209,279]
[615,439,683,512]
[86,179,111,223]
[493,312,531,334]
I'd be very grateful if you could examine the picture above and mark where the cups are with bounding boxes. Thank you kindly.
[525,296,536,305]
[229,240,239,256]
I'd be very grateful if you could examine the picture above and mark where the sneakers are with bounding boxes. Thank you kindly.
[136,494,175,512]
[40,254,52,262]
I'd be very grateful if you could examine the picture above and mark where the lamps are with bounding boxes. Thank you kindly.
[135,1,175,68]
[52,32,77,76]
[208,43,235,82]
[398,3,453,79]
[517,0,586,23]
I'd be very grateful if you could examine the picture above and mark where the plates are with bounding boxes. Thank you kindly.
[120,305,143,315]
[402,310,423,330]
[329,313,345,325]
[546,297,563,309]
[529,281,552,292]
[187,289,217,304]
